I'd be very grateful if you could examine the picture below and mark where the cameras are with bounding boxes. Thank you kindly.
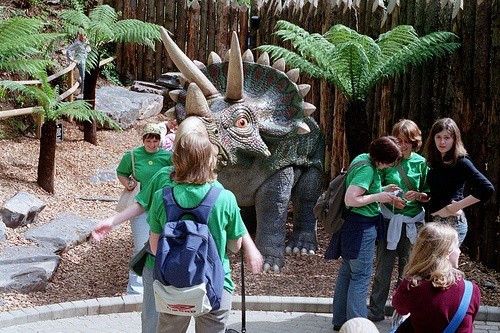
[394,189,403,198]
[128,179,135,187]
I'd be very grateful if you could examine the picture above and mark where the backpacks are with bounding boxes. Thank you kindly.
[312,161,376,235]
[152,186,226,319]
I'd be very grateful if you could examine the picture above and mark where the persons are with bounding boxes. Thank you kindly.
[367,121,432,321]
[390,221,481,333]
[331,136,405,331]
[423,119,494,246]
[146,131,246,333]
[66,32,90,94]
[118,123,175,293]
[92,166,263,333]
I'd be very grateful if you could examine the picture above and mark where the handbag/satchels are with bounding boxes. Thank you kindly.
[115,181,141,215]
[128,241,151,276]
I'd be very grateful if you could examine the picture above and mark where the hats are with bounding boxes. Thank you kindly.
[142,123,161,135]
